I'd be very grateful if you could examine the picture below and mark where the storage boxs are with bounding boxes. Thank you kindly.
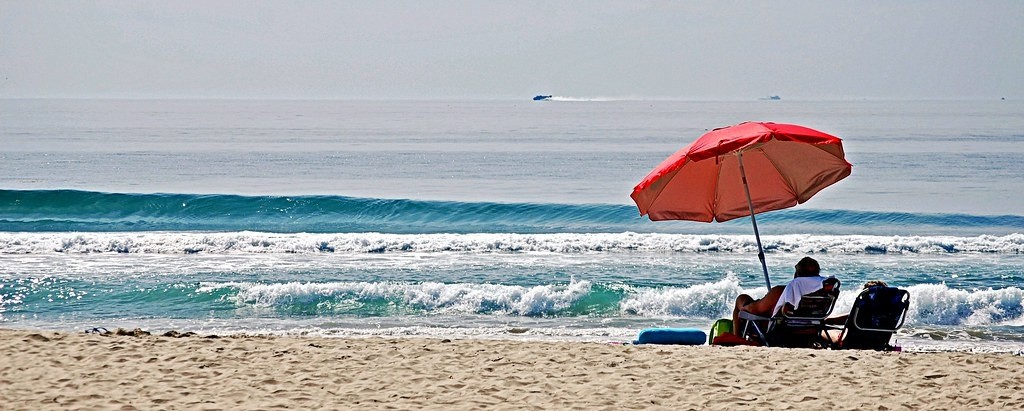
[716,321,733,336]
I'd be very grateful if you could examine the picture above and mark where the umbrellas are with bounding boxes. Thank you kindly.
[631,121,853,293]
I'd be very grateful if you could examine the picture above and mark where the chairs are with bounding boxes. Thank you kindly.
[739,278,840,347]
[817,288,910,349]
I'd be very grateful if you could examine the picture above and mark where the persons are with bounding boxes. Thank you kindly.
[732,256,821,339]
[821,280,890,344]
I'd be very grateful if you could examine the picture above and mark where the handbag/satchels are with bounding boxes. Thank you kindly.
[708,318,733,345]
